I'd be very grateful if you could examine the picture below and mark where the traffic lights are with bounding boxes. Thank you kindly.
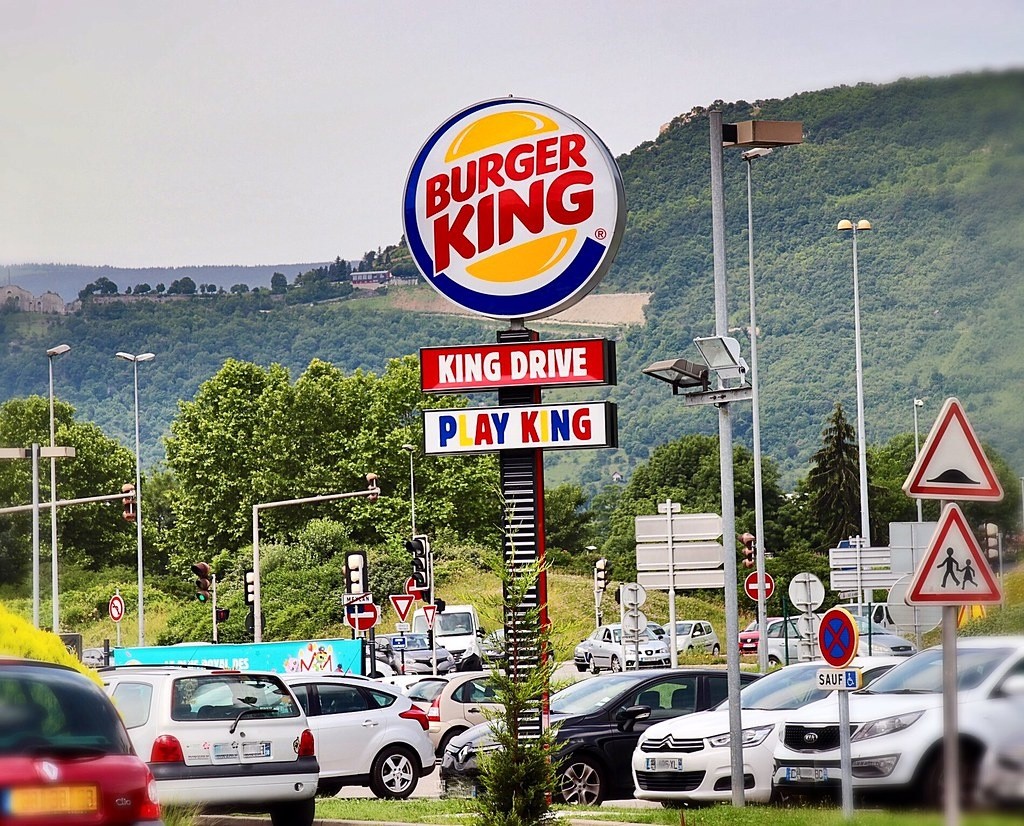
[216,609,230,621]
[405,534,429,590]
[977,522,998,561]
[190,562,210,603]
[595,559,613,589]
[737,532,756,568]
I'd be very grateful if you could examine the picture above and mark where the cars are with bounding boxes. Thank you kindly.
[439,668,764,809]
[573,615,917,674]
[0,659,166,826]
[96,664,320,826]
[185,675,437,802]
[366,605,506,676]
[631,655,908,812]
[81,647,115,666]
[772,635,1024,813]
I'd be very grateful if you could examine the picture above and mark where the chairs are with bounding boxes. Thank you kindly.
[670,689,690,710]
[638,691,665,710]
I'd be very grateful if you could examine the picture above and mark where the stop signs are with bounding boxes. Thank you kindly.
[346,603,378,630]
[744,571,775,602]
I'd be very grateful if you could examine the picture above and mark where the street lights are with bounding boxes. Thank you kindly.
[116,352,155,648]
[741,145,776,675]
[837,219,872,607]
[641,110,805,808]
[0,443,75,632]
[47,343,71,636]
[912,397,925,524]
